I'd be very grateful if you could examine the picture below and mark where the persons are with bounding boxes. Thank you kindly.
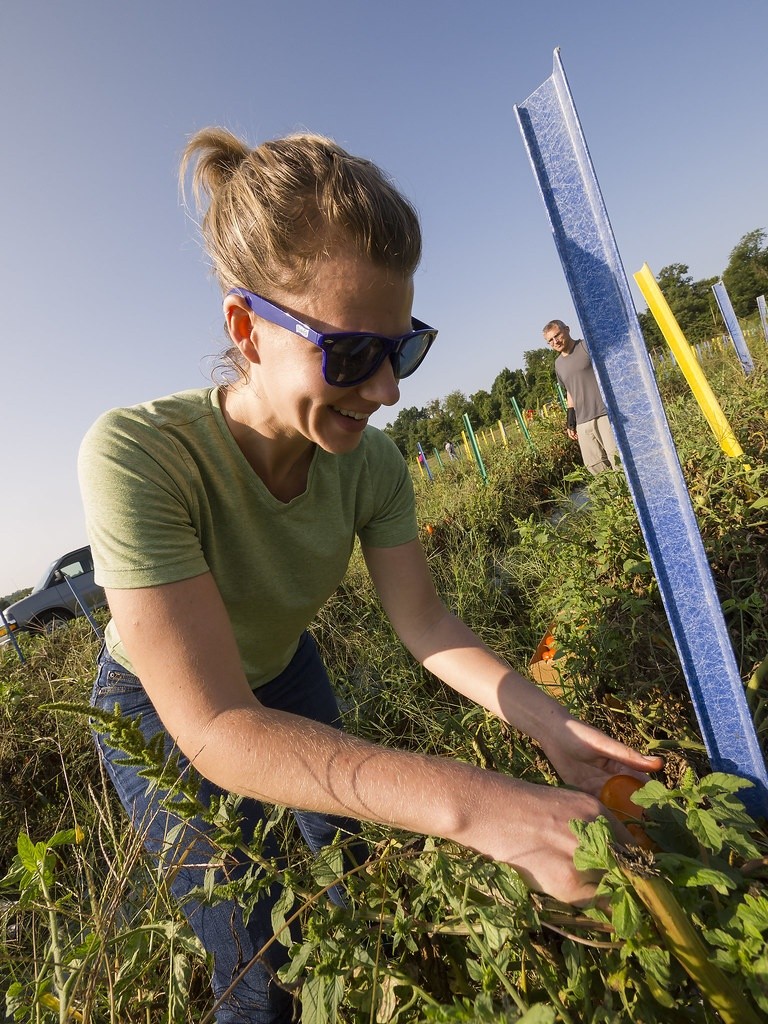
[542,320,622,477]
[444,440,459,461]
[77,134,663,1024]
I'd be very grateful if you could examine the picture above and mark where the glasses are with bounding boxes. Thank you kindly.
[222,288,439,388]
[547,328,565,346]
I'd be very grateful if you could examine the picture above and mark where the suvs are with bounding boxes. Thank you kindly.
[2,543,114,645]
